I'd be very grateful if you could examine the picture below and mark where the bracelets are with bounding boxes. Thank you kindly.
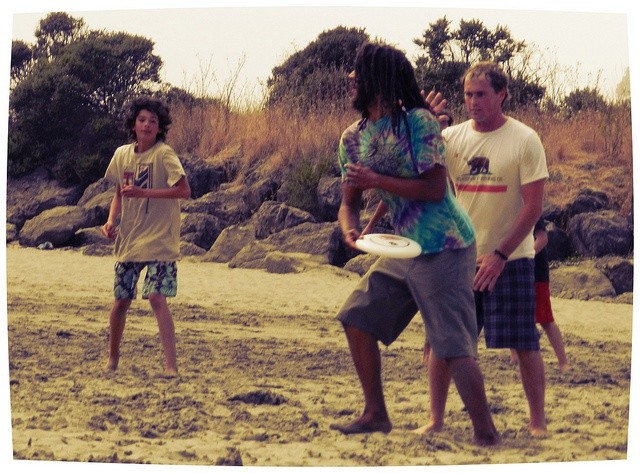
[494,247,508,263]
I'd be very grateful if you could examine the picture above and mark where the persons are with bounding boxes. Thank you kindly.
[329,42,502,446]
[412,61,550,440]
[99,97,191,379]
[356,194,386,237]
[509,213,567,377]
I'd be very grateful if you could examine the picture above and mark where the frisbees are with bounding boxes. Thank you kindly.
[355,233,422,258]
